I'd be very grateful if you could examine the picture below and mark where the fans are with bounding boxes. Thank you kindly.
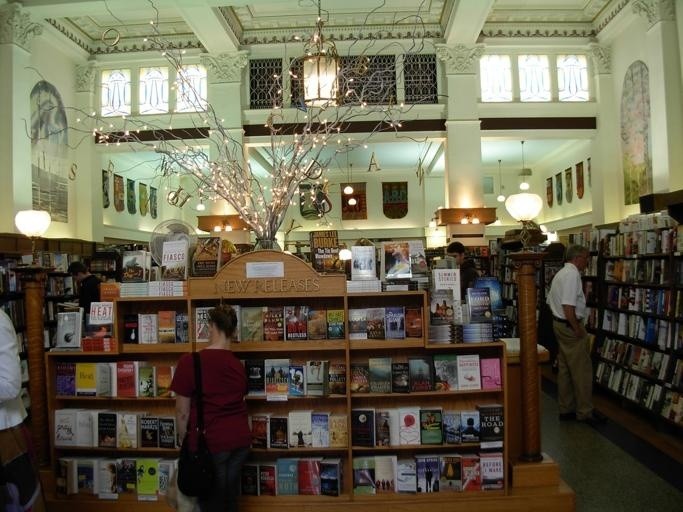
[148,219,199,268]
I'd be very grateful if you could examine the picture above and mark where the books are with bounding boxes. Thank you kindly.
[309,230,431,292]
[0,302,31,433]
[229,269,510,498]
[0,253,83,307]
[53,302,216,499]
[463,234,571,347]
[572,209,683,426]
[92,236,223,302]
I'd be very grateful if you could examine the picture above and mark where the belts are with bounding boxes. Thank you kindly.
[554,318,568,323]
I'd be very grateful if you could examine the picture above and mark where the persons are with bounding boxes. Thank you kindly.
[447,241,480,299]
[67,262,104,312]
[546,245,603,422]
[0,307,39,512]
[168,305,253,512]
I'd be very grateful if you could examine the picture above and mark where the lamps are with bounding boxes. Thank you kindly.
[302,0,340,108]
[14,210,51,269]
[504,193,543,255]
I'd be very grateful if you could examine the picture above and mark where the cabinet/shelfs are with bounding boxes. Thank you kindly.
[0,233,33,421]
[28,236,146,352]
[425,245,596,421]
[596,225,683,492]
[45,248,577,512]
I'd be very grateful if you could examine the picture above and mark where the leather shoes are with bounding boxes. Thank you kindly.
[559,412,608,424]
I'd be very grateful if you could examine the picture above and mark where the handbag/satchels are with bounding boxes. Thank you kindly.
[177,433,218,497]
